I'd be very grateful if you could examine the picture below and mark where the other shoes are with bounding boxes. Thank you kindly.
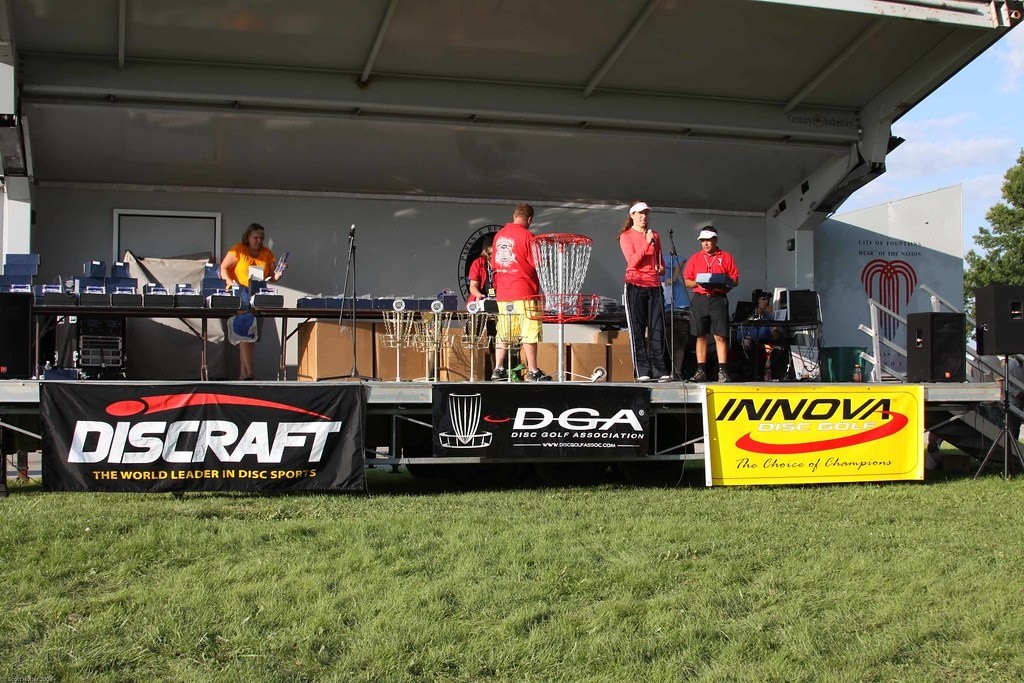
[658,376,671,381]
[636,375,650,380]
[764,376,779,381]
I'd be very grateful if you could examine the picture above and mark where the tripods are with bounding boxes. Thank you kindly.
[633,227,697,382]
[972,354,1024,479]
[317,235,382,382]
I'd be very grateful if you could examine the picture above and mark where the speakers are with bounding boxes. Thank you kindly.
[975,286,1024,354]
[906,311,965,383]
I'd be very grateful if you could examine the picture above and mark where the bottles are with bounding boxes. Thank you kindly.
[852,364,862,382]
[274,252,289,276]
[802,365,809,380]
[233,279,249,302]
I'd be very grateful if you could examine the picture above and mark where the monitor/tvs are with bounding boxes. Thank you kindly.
[771,288,787,320]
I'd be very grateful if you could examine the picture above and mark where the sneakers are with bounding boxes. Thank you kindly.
[718,369,730,382]
[491,368,508,381]
[690,368,707,381]
[527,367,552,381]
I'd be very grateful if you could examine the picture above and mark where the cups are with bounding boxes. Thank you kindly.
[437,287,451,299]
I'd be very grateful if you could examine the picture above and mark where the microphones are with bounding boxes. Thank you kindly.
[347,224,357,243]
[646,228,655,245]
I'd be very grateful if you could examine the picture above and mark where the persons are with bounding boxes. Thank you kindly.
[661,254,691,381]
[220,223,288,380]
[616,200,672,382]
[465,237,497,373]
[491,203,554,381]
[682,226,739,383]
[736,289,790,382]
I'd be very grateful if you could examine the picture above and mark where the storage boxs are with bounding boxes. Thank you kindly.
[0,254,241,309]
[517,329,633,382]
[819,346,868,383]
[251,288,457,311]
[297,321,507,381]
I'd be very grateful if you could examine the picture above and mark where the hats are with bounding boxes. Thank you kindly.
[752,288,773,299]
[629,202,651,213]
[697,230,718,239]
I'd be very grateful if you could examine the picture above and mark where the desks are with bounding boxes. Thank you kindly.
[729,321,823,382]
[259,308,490,381]
[33,306,260,382]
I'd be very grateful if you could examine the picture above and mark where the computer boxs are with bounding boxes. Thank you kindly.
[779,288,818,323]
